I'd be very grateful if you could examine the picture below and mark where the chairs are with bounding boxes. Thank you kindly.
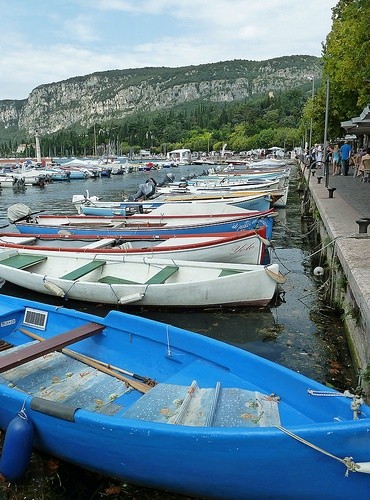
[349,154,370,183]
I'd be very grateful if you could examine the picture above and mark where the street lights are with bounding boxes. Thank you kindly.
[305,73,314,165]
[313,60,330,176]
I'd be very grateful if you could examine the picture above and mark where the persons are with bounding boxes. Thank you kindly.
[306,140,370,182]
[340,140,351,176]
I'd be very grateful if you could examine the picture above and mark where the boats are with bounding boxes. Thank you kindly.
[0,293,370,500]
[0,154,290,308]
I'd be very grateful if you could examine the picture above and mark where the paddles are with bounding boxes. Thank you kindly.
[19,329,154,394]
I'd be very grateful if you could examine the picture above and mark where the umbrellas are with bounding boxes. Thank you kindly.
[267,147,282,159]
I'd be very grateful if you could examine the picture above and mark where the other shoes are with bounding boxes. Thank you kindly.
[341,173,348,176]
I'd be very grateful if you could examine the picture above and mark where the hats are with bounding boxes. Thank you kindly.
[344,141,348,143]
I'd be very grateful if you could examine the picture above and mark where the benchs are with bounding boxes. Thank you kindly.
[0,255,47,269]
[60,260,106,281]
[108,222,125,228]
[82,239,116,248]
[0,323,106,373]
[144,266,180,284]
